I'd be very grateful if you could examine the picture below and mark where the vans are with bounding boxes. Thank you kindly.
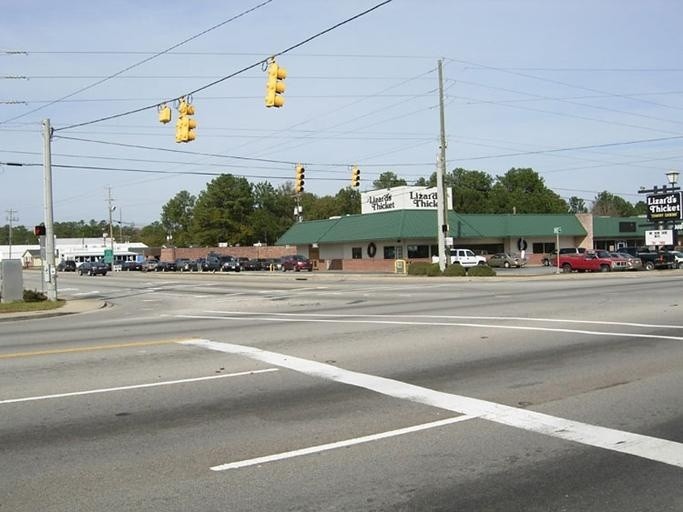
[541,247,586,266]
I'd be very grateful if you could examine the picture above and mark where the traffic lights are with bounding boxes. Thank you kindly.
[352,166,361,187]
[295,161,305,194]
[35,225,46,236]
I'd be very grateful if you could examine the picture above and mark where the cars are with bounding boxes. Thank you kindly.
[431,249,487,269]
[100,251,313,273]
[611,247,683,273]
[77,262,110,276]
[56,260,76,272]
[486,252,528,268]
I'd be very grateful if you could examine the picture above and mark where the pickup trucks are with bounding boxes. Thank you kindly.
[554,250,627,274]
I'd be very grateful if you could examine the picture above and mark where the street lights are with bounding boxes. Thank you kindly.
[664,169,680,250]
